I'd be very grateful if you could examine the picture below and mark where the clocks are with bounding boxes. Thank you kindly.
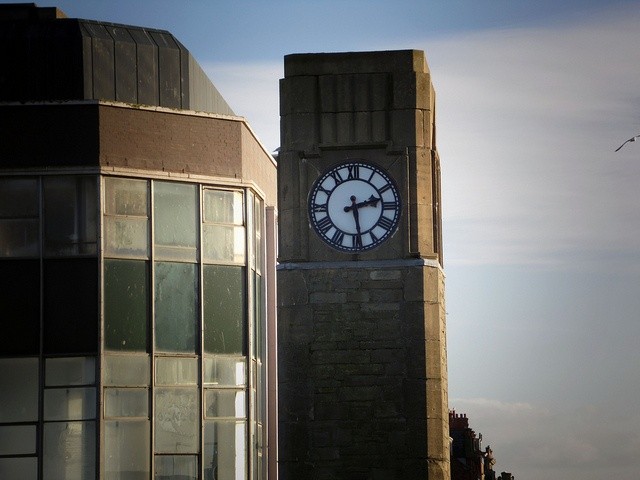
[296,140,411,260]
